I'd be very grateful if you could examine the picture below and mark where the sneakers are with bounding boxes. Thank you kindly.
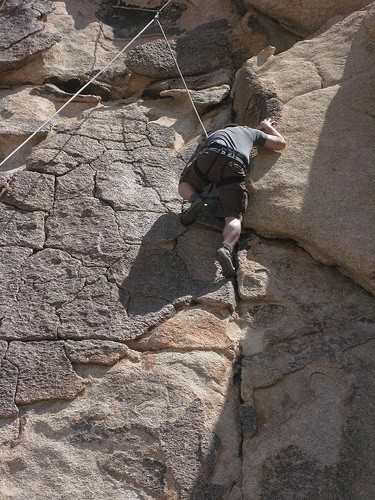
[180,198,205,225]
[215,246,237,277]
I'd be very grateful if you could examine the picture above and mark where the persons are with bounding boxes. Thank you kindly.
[178,116,287,280]
